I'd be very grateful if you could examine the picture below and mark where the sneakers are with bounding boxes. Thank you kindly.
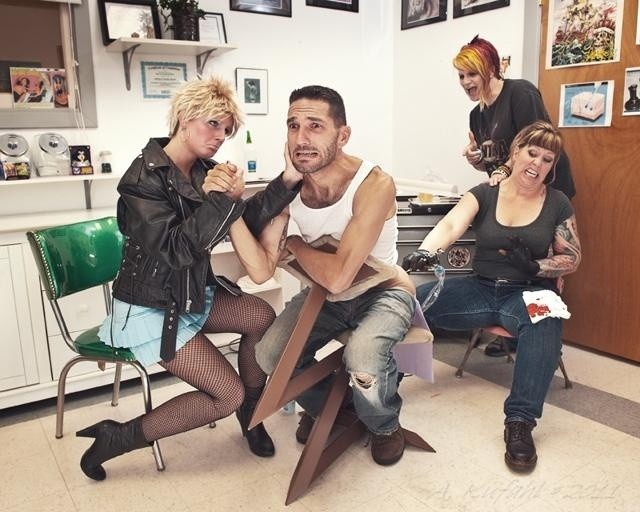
[503,411,540,472]
[371,429,406,465]
[485,334,520,357]
[295,386,356,444]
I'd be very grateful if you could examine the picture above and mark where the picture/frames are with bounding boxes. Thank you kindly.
[451,0,513,22]
[94,0,162,47]
[228,0,293,20]
[302,0,361,15]
[398,0,448,33]
[193,11,226,44]
[232,64,272,117]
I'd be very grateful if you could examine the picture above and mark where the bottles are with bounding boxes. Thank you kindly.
[625,84,640,112]
[98,150,113,173]
[242,131,260,175]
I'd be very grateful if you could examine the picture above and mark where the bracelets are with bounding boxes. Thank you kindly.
[491,166,512,176]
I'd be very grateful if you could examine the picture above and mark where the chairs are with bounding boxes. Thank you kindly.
[242,238,439,507]
[455,246,575,393]
[23,212,219,476]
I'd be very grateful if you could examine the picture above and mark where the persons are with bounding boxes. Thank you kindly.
[75,73,303,481]
[202,85,416,465]
[402,120,581,471]
[71,148,88,174]
[453,35,577,355]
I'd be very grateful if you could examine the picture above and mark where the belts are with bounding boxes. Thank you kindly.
[480,275,538,287]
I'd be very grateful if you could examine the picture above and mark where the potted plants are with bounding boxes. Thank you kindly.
[155,0,209,40]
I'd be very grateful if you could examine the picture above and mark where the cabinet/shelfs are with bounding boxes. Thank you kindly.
[32,218,164,395]
[0,224,48,413]
[205,183,300,360]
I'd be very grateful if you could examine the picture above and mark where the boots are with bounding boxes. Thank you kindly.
[76,414,156,481]
[236,384,278,458]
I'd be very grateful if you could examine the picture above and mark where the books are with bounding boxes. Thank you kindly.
[68,145,94,176]
[10,67,68,108]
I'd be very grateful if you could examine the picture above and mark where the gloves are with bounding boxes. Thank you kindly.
[400,248,441,275]
[500,233,540,276]
